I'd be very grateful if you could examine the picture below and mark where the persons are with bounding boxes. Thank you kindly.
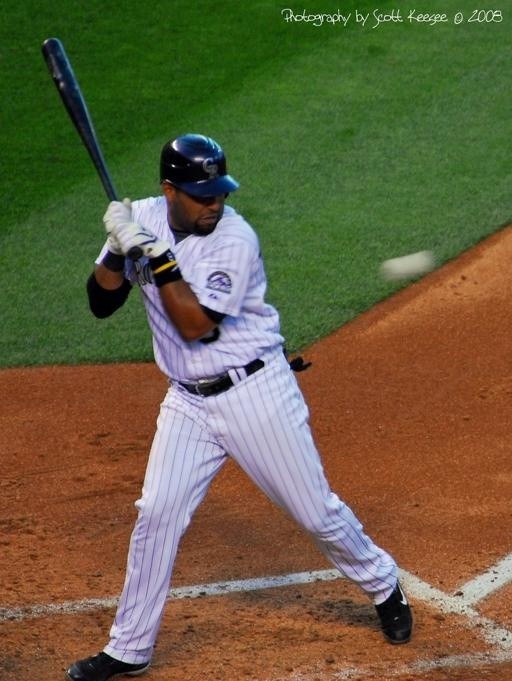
[67,134,414,681]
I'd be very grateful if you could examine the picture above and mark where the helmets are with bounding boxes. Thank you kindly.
[160,134,240,198]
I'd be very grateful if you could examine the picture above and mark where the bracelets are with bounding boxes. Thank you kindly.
[103,251,125,272]
[148,248,183,288]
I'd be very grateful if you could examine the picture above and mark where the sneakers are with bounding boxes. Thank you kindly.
[64,651,150,681]
[375,578,412,644]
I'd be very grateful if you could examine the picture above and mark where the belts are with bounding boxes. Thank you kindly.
[179,359,265,398]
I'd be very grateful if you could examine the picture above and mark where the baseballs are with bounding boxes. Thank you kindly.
[381,249,435,285]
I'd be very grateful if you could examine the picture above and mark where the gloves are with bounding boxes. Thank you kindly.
[102,197,133,255]
[112,223,169,258]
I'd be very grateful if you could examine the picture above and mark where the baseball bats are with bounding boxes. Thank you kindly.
[42,39,143,260]
